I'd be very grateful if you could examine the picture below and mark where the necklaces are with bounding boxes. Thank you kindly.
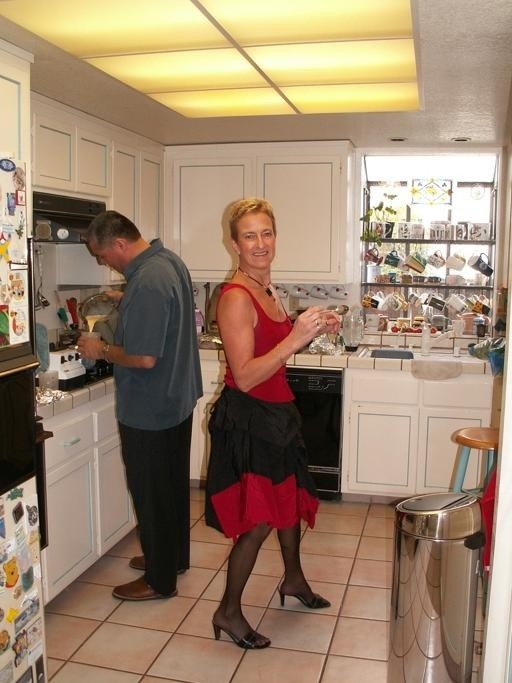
[238,266,272,296]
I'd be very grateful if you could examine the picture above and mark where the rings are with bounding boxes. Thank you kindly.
[316,320,320,326]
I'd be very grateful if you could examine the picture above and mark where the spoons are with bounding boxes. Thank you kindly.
[37,250,50,308]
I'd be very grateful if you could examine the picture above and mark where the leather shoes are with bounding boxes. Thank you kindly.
[111,572,179,601]
[128,553,188,575]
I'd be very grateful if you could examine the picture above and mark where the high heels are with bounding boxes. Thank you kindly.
[278,587,332,609]
[211,618,271,650]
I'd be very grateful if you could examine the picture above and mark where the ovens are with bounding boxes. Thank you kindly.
[284,367,344,502]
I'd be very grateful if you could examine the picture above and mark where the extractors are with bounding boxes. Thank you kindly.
[33,192,108,245]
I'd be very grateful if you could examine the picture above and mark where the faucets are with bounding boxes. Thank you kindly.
[420,322,456,356]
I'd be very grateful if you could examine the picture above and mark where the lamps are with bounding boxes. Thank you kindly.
[0,1,424,118]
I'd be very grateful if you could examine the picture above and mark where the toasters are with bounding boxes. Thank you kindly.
[44,342,85,390]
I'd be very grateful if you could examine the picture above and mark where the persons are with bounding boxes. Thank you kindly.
[77,210,203,601]
[204,198,341,649]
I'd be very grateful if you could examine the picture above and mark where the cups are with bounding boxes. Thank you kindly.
[365,313,380,335]
[385,292,401,311]
[361,292,379,309]
[79,293,120,344]
[446,294,468,313]
[396,293,408,311]
[388,273,397,284]
[38,370,59,391]
[364,247,383,266]
[427,277,442,285]
[401,274,411,283]
[408,292,419,307]
[472,295,490,315]
[310,284,329,299]
[452,320,466,336]
[274,284,288,298]
[371,221,392,239]
[427,292,446,311]
[290,284,310,299]
[428,250,445,268]
[405,252,425,273]
[446,254,466,270]
[329,285,350,299]
[412,276,427,284]
[372,290,388,311]
[79,332,99,368]
[468,252,493,277]
[384,251,399,267]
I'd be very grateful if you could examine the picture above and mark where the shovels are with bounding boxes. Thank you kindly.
[34,248,51,309]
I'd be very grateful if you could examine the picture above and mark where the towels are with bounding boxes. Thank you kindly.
[410,358,463,381]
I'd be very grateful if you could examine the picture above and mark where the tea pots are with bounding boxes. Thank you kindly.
[338,305,364,351]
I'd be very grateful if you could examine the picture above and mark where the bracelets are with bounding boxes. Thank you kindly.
[101,345,110,365]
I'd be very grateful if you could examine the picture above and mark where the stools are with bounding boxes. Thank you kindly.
[452,426,499,498]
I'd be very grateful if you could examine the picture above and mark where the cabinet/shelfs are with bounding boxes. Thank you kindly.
[57,138,165,287]
[39,397,137,603]
[165,140,357,285]
[190,359,229,487]
[33,114,112,201]
[1,38,33,239]
[362,185,497,356]
[342,365,493,506]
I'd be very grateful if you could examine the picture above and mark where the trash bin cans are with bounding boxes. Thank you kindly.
[385,492,484,678]
